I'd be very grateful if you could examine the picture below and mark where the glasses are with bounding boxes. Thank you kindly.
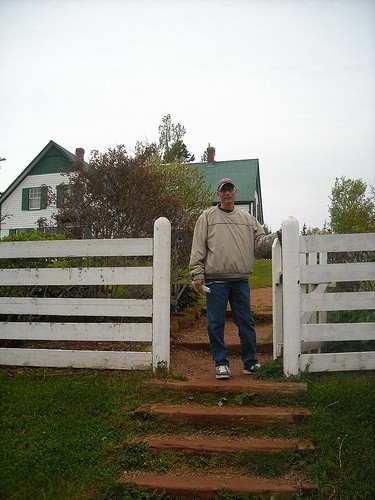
[218,188,235,193]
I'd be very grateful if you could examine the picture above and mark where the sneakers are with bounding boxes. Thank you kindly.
[243,363,261,374]
[215,365,231,379]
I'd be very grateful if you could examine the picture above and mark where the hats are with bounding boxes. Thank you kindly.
[216,178,236,192]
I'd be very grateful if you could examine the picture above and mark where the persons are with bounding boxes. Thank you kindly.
[189,178,282,379]
